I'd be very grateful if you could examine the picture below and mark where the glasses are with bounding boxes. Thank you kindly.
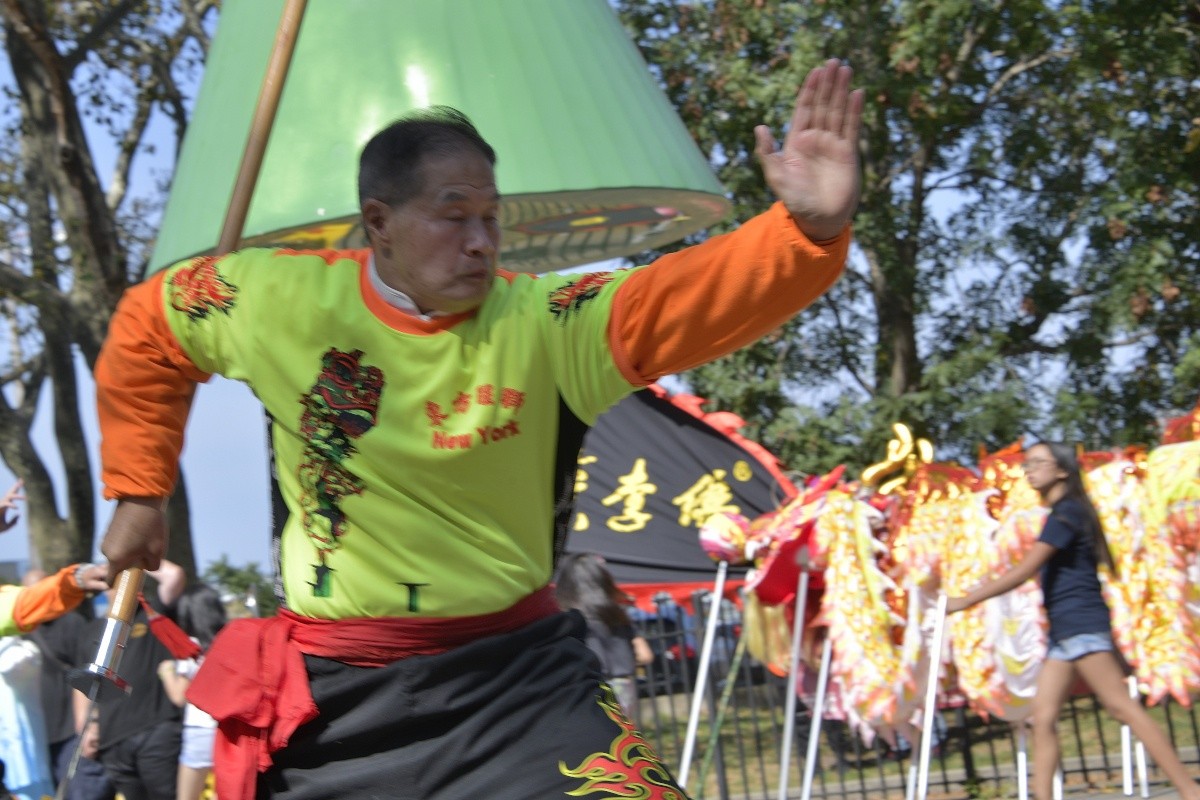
[1021,457,1057,469]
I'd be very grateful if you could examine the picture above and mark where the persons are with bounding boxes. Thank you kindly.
[557,552,650,735]
[936,440,1200,800]
[1,477,233,800]
[97,56,867,800]
